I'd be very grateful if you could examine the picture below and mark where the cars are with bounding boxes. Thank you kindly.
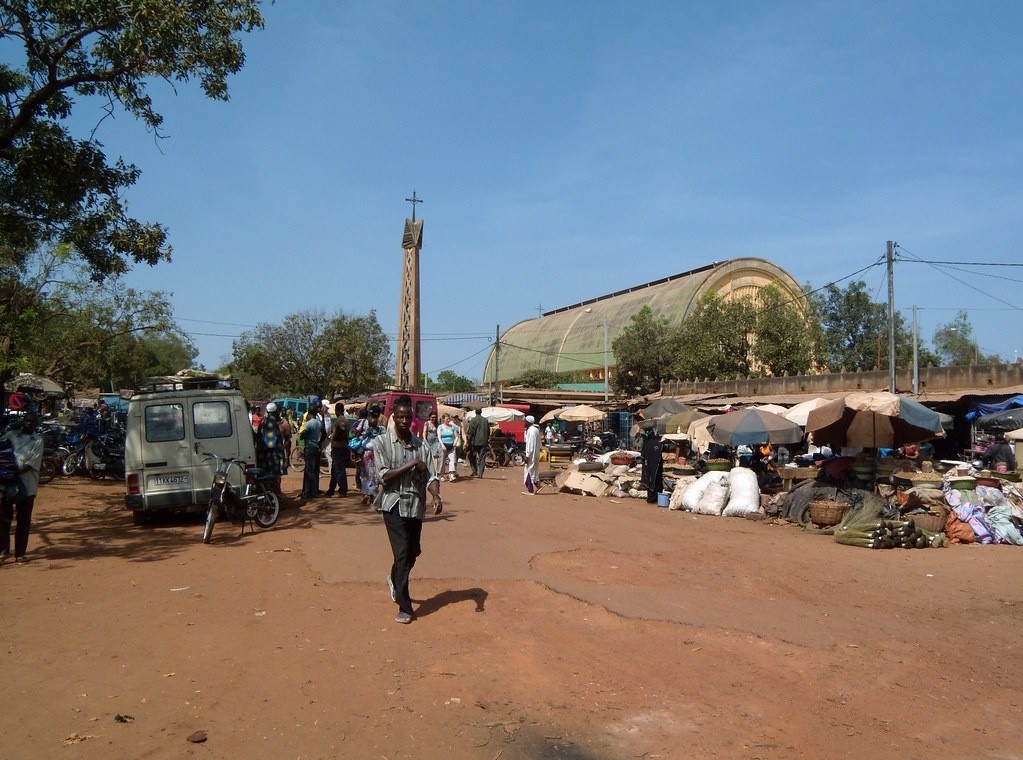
[295,415,362,468]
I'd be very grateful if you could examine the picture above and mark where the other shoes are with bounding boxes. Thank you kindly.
[332,492,349,498]
[440,476,445,482]
[521,491,534,495]
[536,484,546,493]
[16,556,31,562]
[449,473,457,481]
[478,475,482,478]
[0,551,11,562]
[470,473,478,477]
[301,493,313,499]
[361,497,370,504]
[322,494,332,498]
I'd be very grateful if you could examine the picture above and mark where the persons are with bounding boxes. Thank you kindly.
[504,440,518,460]
[521,415,545,496]
[244,400,297,522]
[371,401,443,624]
[297,395,412,508]
[544,420,559,438]
[423,411,463,482]
[467,407,490,478]
[539,442,549,462]
[0,384,112,562]
[971,434,1014,471]
[639,427,664,504]
[592,426,935,494]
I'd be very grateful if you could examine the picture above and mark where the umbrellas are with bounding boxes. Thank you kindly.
[965,395,1023,440]
[4,373,65,396]
[628,391,953,495]
[539,404,608,422]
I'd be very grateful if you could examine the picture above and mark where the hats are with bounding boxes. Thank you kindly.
[266,403,277,412]
[451,413,458,417]
[321,399,332,408]
[525,415,535,423]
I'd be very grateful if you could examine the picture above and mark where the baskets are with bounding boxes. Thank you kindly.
[905,511,949,532]
[809,501,852,526]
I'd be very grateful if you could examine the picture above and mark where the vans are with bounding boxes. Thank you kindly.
[124,378,258,524]
[272,398,309,418]
[365,386,437,439]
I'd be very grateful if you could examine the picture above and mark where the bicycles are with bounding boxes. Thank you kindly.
[289,443,330,474]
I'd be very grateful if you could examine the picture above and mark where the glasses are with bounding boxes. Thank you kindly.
[453,417,458,418]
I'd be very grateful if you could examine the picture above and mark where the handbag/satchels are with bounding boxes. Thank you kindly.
[444,443,453,451]
[348,436,362,452]
[5,478,27,503]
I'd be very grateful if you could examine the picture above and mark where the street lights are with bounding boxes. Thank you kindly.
[585,307,609,401]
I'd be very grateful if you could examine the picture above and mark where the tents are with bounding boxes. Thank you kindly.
[437,401,465,419]
[465,406,525,425]
[329,395,367,414]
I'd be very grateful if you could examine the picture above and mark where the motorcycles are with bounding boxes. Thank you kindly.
[202,452,281,542]
[581,430,618,462]
[61,417,125,481]
[487,430,526,466]
[0,423,66,486]
